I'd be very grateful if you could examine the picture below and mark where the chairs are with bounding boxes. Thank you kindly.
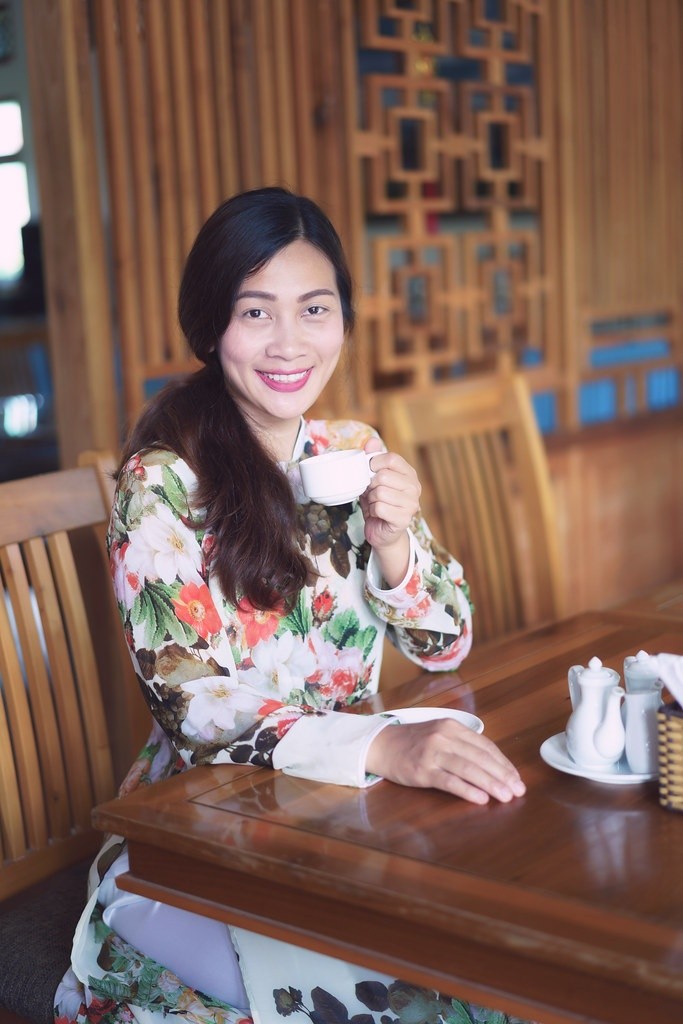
[0,326,569,1024]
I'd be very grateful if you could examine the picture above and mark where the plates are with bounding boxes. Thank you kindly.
[539,727,661,785]
[376,706,485,737]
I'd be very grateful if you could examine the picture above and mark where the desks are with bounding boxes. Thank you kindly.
[91,609,683,1024]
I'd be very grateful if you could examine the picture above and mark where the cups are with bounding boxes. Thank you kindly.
[299,448,384,506]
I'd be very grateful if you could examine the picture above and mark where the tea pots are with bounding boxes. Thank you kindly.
[619,649,668,737]
[564,656,627,770]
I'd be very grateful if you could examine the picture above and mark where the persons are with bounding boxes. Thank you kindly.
[53,185,527,1024]
